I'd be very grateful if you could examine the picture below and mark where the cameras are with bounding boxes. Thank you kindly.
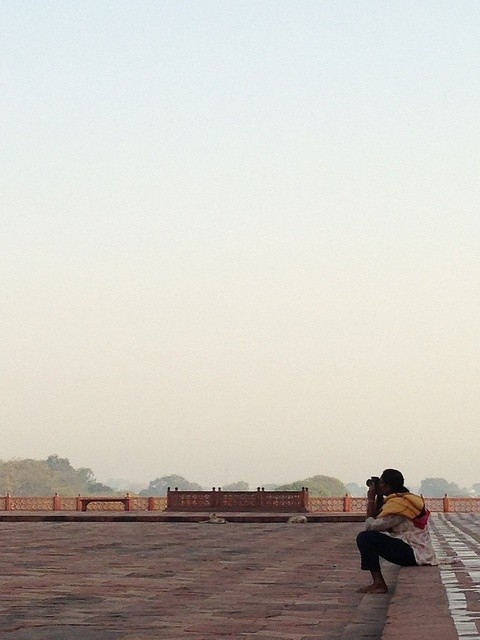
[366,477,382,494]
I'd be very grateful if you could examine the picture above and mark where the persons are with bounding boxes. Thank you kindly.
[355,469,437,594]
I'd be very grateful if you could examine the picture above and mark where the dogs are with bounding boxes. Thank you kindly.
[288,516,307,524]
[198,513,229,525]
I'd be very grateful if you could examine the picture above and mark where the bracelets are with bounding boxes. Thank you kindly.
[367,499,375,504]
